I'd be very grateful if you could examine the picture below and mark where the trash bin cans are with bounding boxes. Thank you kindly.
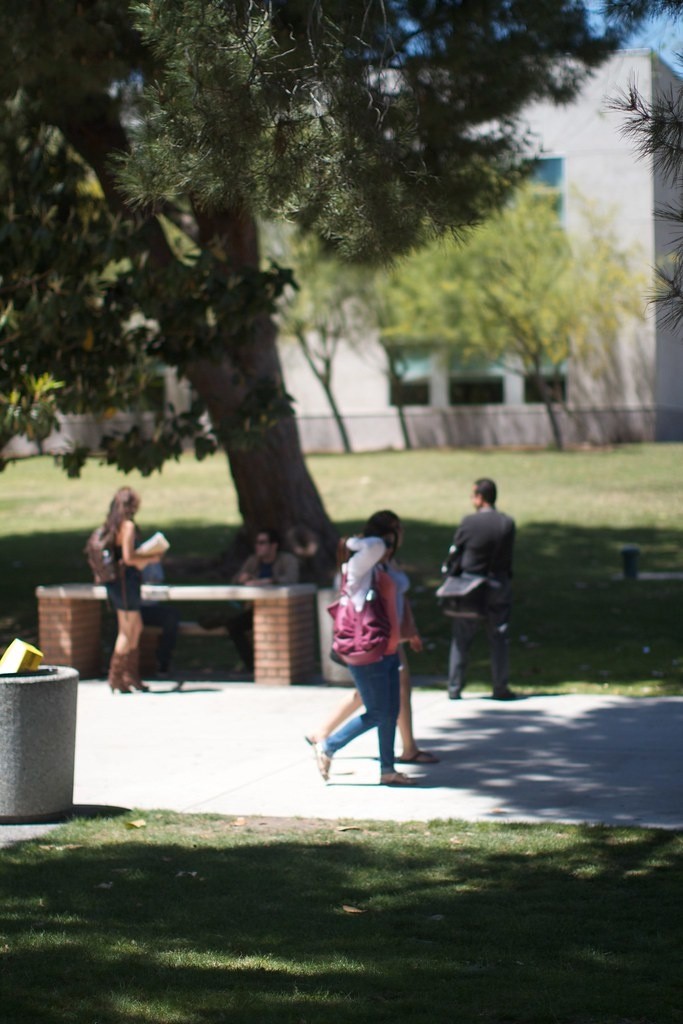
[0,664,79,826]
[317,588,356,687]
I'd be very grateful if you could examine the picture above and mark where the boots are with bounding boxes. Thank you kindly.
[108,650,132,694]
[118,648,149,693]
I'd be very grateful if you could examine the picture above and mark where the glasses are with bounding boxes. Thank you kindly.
[256,538,271,546]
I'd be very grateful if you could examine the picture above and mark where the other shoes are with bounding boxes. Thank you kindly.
[492,689,515,700]
[448,691,462,700]
[229,662,255,679]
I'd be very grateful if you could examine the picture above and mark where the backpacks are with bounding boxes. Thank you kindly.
[85,527,119,584]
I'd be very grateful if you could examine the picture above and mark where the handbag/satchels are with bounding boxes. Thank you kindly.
[436,569,501,618]
[327,570,391,665]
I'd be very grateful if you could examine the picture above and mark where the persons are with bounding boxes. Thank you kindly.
[306,510,440,785]
[225,528,299,672]
[442,478,517,701]
[87,487,160,693]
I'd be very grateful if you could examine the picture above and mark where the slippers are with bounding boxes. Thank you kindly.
[314,744,332,780]
[396,749,440,764]
[379,771,420,788]
[305,735,317,753]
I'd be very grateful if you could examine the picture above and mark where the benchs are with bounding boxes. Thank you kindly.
[139,623,251,679]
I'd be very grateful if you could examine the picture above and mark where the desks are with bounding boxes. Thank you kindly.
[36,581,319,686]
